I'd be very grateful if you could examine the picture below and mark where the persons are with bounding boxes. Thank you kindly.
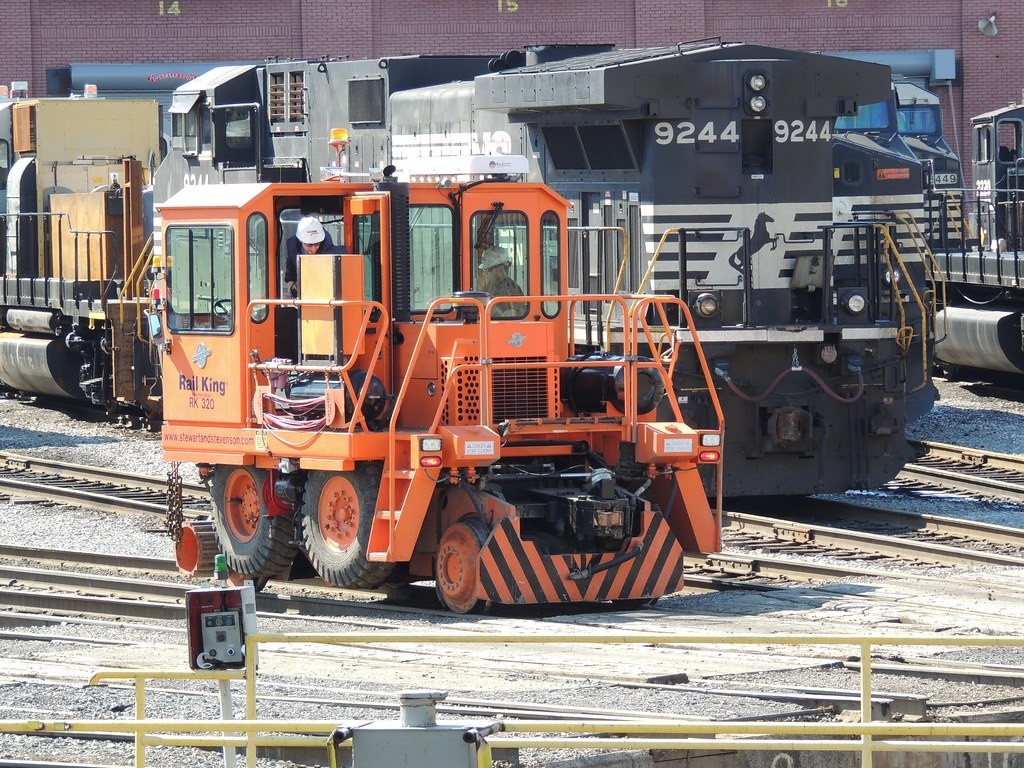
[479,245,525,316]
[284,215,335,299]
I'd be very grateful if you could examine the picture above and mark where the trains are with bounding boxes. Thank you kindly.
[0,31,951,517]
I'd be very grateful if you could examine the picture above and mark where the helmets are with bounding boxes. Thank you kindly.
[296,216,325,243]
[479,246,510,269]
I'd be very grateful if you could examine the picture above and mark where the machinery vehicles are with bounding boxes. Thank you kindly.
[118,164,727,616]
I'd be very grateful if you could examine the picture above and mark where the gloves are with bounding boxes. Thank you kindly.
[288,281,298,298]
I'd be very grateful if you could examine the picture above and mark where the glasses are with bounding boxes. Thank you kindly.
[304,242,320,247]
[481,267,498,272]
